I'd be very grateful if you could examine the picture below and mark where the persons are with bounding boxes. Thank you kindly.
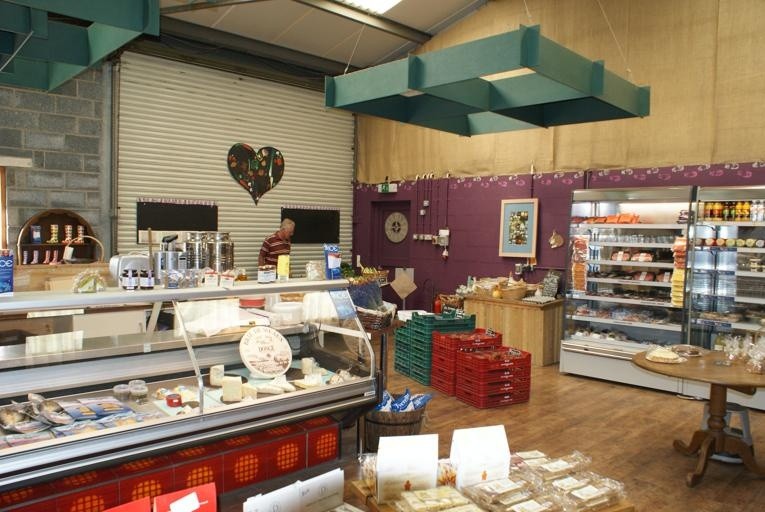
[258,218,296,278]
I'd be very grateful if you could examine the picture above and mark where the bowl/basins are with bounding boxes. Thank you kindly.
[729,305,748,313]
[746,315,761,323]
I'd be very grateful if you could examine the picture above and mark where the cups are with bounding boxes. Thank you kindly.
[278,254,289,282]
[306,261,323,281]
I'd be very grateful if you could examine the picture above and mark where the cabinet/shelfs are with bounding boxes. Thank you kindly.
[688,184,765,411]
[16,206,100,266]
[0,276,378,510]
[560,184,690,393]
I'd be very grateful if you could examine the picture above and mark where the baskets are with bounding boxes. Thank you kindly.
[394,309,531,409]
[502,284,527,299]
[354,300,397,331]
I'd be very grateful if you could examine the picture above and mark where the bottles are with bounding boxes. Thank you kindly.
[140,269,155,289]
[258,266,270,283]
[467,276,473,292]
[121,269,139,290]
[714,333,724,351]
[265,265,276,282]
[113,380,148,403]
[535,288,542,296]
[695,199,765,247]
[382,176,389,192]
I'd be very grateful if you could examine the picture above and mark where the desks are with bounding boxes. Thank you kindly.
[629,347,765,487]
[463,295,563,367]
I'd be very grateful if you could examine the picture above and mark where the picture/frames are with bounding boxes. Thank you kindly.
[498,198,539,258]
